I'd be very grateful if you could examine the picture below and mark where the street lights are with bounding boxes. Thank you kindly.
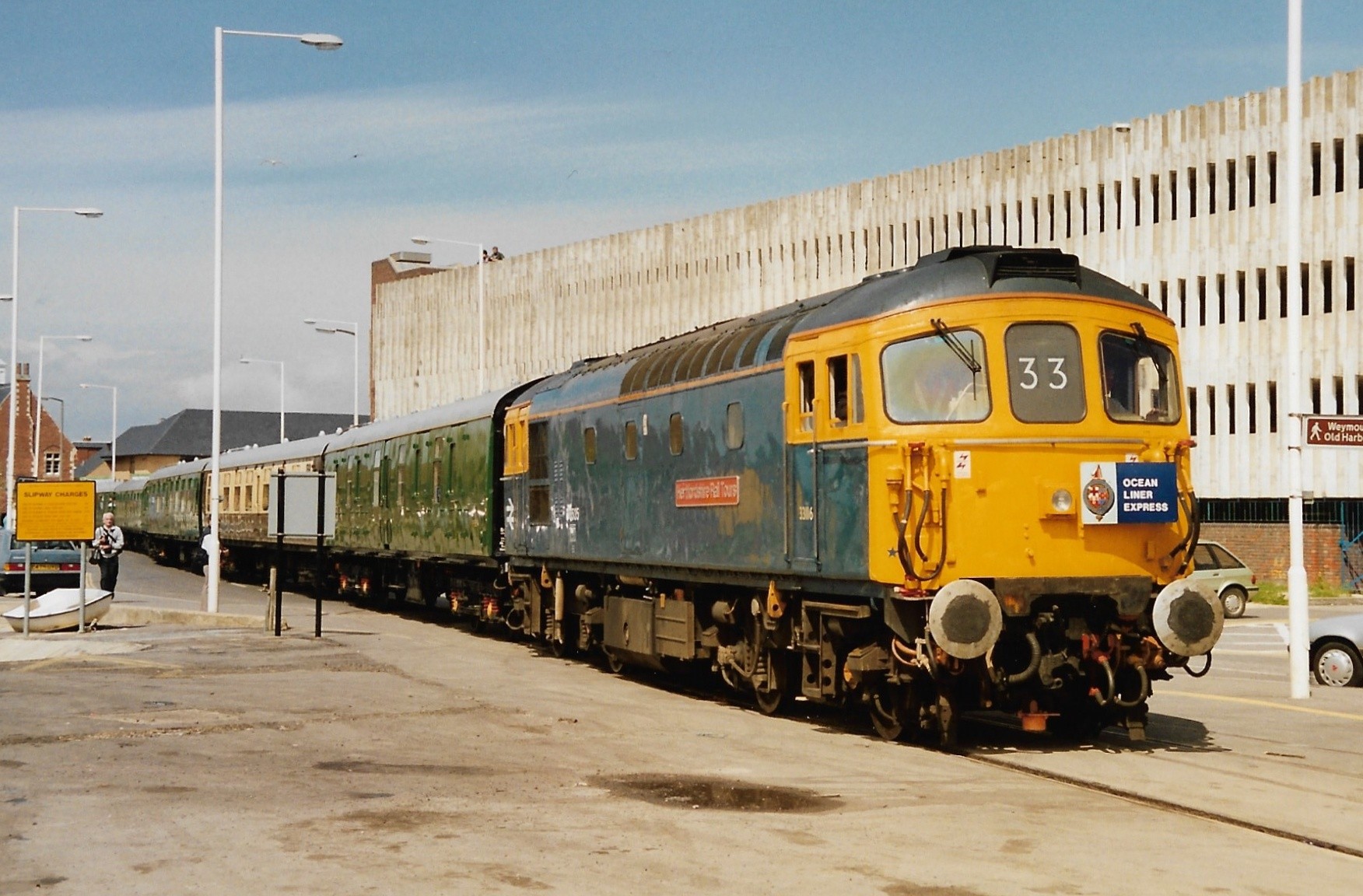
[202,28,344,616]
[303,319,359,427]
[240,359,284,443]
[411,237,484,396]
[41,396,63,481]
[33,335,93,479]
[7,207,104,530]
[80,383,116,480]
[1115,123,1132,287]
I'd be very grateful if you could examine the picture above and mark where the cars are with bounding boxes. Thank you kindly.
[1288,614,1363,688]
[0,534,80,597]
[1182,541,1259,619]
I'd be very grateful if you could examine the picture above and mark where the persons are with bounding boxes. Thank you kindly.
[199,514,229,612]
[92,512,123,598]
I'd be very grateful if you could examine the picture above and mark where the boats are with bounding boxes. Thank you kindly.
[1,588,114,633]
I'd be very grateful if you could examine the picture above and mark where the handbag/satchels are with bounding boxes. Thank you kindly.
[89,546,101,565]
[117,547,122,554]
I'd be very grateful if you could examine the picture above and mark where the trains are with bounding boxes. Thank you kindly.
[108,244,1223,756]
